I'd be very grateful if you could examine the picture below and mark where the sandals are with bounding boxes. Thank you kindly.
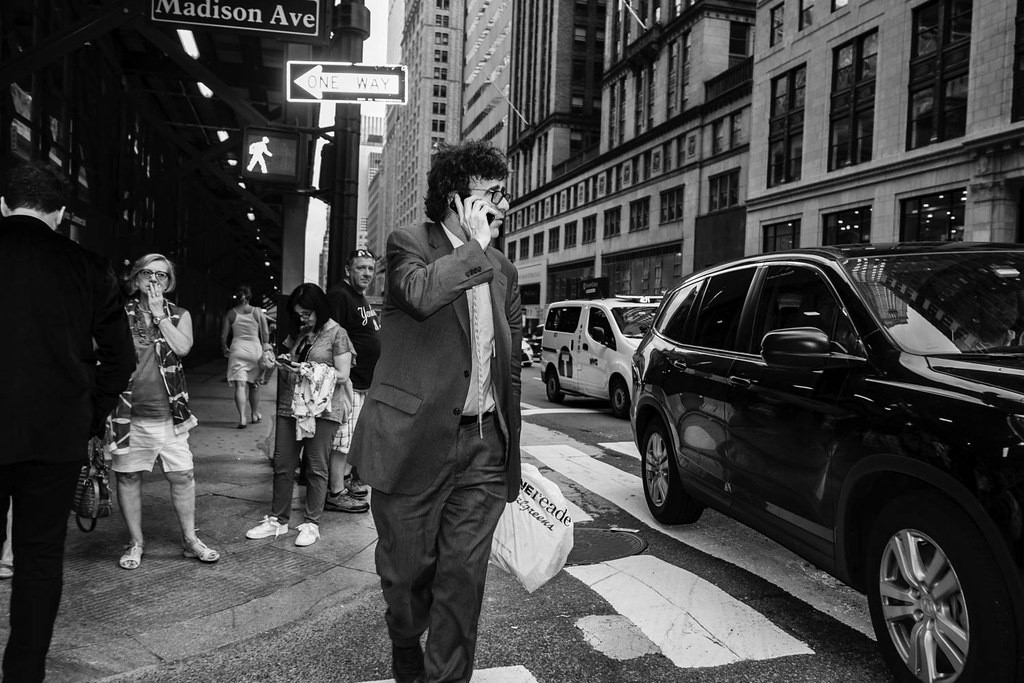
[120,542,143,569]
[180,535,220,562]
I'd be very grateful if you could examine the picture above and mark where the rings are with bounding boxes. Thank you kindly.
[151,293,160,297]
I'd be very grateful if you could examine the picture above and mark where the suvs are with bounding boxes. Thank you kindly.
[629,240,1024,683]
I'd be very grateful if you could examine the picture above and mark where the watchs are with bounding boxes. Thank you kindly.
[153,315,167,324]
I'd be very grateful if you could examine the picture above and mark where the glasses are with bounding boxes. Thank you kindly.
[356,250,373,257]
[472,188,511,204]
[300,310,314,320]
[139,268,167,281]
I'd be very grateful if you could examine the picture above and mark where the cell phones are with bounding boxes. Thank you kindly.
[449,189,495,225]
[275,358,297,368]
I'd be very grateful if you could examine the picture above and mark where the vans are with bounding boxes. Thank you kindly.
[540,295,663,421]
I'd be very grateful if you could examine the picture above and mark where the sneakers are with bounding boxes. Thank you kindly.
[294,523,321,546]
[247,514,288,539]
[327,489,369,512]
[345,478,369,497]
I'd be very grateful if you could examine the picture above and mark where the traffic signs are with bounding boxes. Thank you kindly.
[286,61,409,106]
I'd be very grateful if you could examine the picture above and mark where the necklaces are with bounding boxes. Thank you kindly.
[137,303,152,314]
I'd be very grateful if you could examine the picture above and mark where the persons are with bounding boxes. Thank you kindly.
[0,160,140,683]
[101,253,219,568]
[221,248,383,545]
[345,136,574,683]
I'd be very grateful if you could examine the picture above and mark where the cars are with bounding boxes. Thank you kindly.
[518,320,546,366]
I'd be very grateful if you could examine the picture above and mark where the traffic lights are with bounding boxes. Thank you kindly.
[238,123,318,195]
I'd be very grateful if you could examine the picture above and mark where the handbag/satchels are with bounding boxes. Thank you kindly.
[491,462,593,595]
[260,344,276,367]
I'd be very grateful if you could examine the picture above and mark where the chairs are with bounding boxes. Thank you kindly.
[778,284,826,329]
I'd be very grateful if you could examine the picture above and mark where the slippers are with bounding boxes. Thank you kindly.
[239,424,247,429]
[252,415,262,423]
